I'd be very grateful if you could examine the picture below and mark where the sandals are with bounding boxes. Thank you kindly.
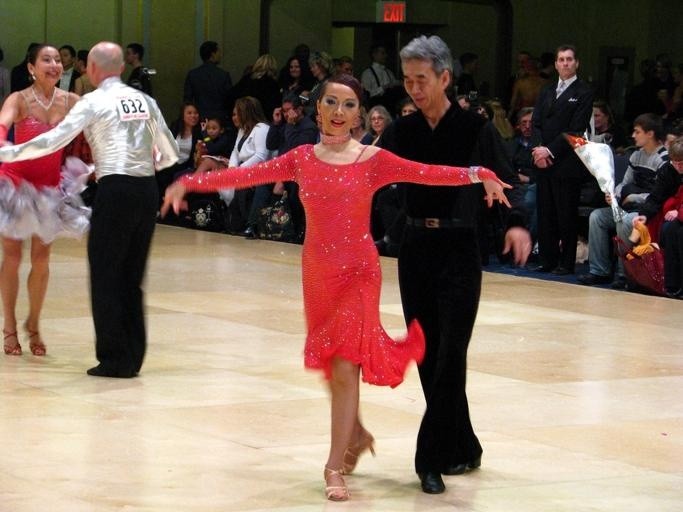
[2,328,23,355]
[22,318,46,356]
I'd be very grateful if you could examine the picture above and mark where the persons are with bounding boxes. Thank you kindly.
[449,41,682,299]
[0,42,92,356]
[374,36,533,494]
[159,42,417,254]
[161,73,515,501]
[0,41,179,378]
[7,42,151,208]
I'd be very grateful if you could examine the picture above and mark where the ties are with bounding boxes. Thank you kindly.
[556,81,565,96]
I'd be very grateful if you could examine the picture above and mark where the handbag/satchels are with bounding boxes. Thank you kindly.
[612,236,667,296]
[188,194,227,232]
[247,189,304,244]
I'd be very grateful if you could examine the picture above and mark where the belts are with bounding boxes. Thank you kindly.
[406,215,470,229]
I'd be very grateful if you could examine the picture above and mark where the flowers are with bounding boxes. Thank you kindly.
[562,132,622,222]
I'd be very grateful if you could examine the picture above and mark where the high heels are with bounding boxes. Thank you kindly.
[323,426,376,501]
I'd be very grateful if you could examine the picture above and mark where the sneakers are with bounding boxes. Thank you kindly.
[609,279,638,291]
[576,272,616,285]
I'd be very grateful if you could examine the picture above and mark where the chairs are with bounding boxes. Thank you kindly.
[578,144,631,218]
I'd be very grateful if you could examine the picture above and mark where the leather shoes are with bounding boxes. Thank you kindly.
[86,363,140,378]
[418,472,445,495]
[442,455,481,475]
[531,262,575,275]
